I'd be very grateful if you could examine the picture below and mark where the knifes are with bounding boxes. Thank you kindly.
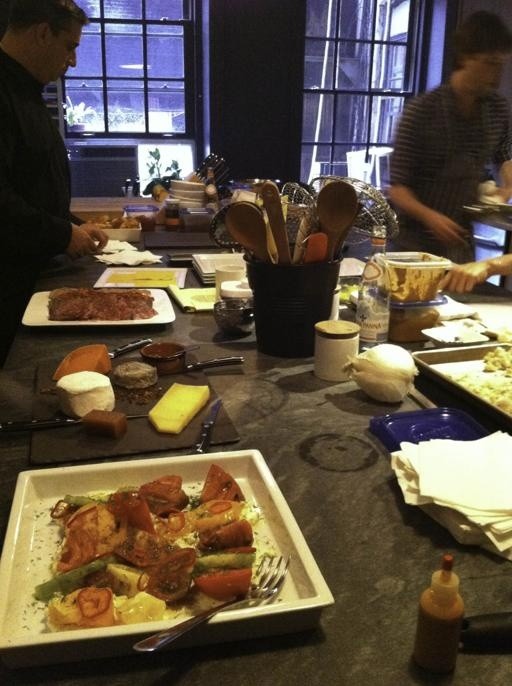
[0,410,150,433]
[195,153,233,185]
[189,398,223,454]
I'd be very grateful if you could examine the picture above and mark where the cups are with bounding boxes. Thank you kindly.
[313,319,362,383]
[214,264,245,301]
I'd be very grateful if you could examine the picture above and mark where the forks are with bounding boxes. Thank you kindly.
[133,553,291,653]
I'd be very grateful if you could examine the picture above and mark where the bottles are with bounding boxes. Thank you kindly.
[355,225,393,353]
[413,555,466,675]
[164,199,180,233]
[205,166,221,214]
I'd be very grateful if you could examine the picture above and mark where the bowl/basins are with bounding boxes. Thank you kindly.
[213,300,255,337]
[168,180,207,209]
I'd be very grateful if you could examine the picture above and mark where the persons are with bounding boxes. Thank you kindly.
[383,10,511,267]
[1,0,110,365]
[434,137,511,304]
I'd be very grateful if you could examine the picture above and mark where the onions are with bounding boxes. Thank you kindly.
[341,344,419,403]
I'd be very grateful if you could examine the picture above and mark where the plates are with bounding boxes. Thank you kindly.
[0,448,336,671]
[433,294,475,323]
[20,289,177,327]
[338,257,367,279]
[191,252,248,284]
[92,266,189,290]
[421,324,492,349]
